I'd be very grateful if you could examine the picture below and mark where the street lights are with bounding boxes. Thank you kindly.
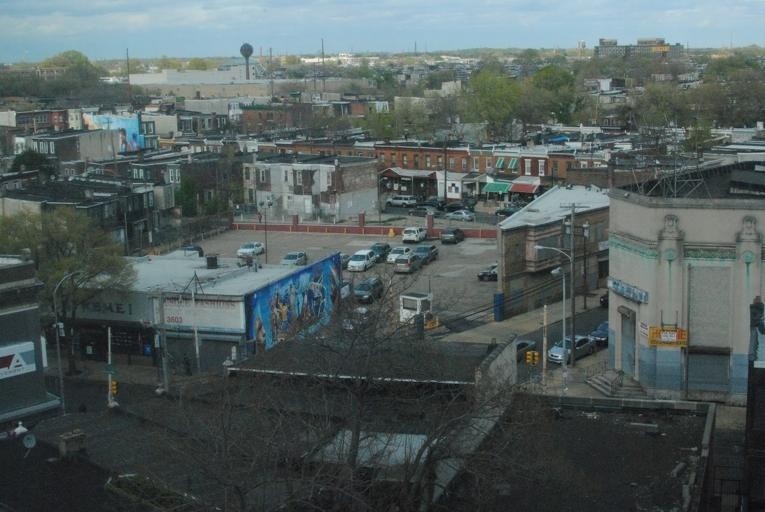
[532,244,578,368]
[65,166,131,256]
[50,268,86,421]
[549,264,569,393]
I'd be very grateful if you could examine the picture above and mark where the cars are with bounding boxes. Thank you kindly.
[386,246,411,264]
[442,227,465,245]
[281,251,307,268]
[494,207,519,217]
[340,252,353,269]
[370,241,391,260]
[346,250,376,273]
[407,205,442,219]
[519,120,670,154]
[237,241,265,257]
[511,292,609,368]
[477,263,500,283]
[416,244,441,262]
[395,253,422,274]
[446,210,475,222]
[421,196,477,211]
[403,226,427,243]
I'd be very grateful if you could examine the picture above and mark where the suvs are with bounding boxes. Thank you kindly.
[386,195,417,208]
[354,278,385,303]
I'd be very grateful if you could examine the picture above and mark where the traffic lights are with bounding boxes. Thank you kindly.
[111,379,121,397]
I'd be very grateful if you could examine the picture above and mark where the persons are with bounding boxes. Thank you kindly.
[132,133,140,152]
[119,127,133,152]
[747,295,765,366]
[14,421,28,436]
